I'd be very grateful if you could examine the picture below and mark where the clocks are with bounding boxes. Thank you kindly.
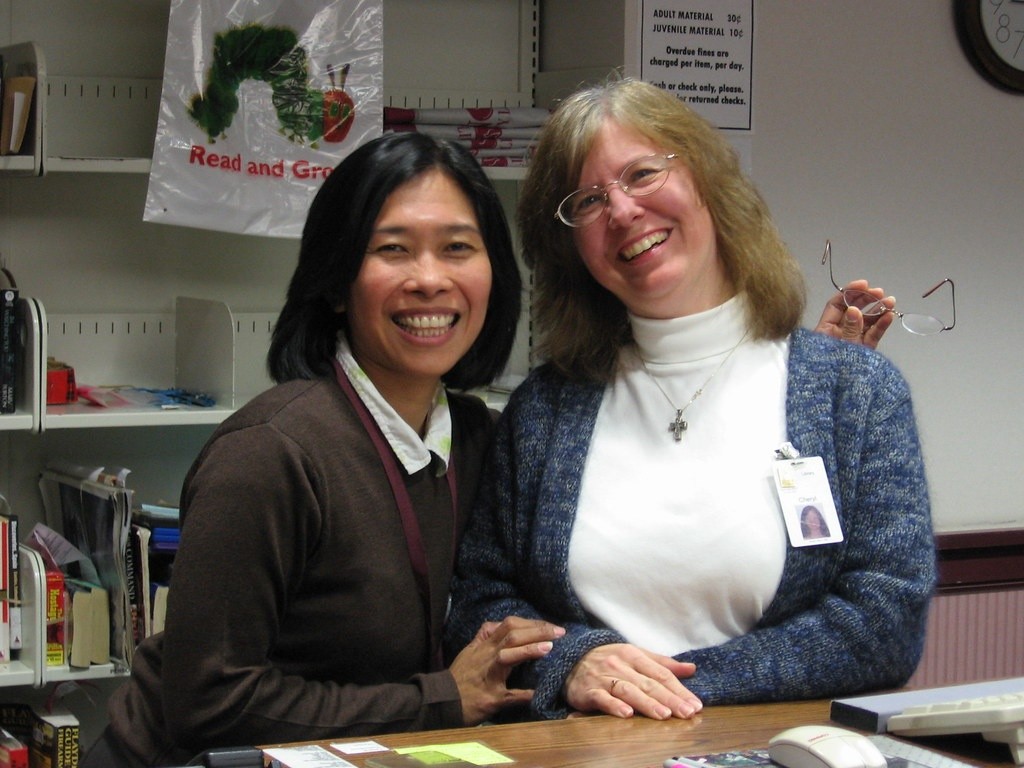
[953,0,1024,95]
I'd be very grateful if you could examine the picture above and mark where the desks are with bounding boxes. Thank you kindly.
[255,700,1024,767]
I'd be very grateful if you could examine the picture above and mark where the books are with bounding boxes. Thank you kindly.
[1,288,19,416]
[0,460,180,670]
[0,697,79,768]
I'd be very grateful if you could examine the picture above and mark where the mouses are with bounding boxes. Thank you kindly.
[769,724,888,768]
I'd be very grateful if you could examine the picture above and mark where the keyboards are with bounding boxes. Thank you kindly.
[886,691,1024,766]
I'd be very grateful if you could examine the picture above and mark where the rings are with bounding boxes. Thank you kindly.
[609,679,625,695]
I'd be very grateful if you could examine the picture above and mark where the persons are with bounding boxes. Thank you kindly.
[443,76,933,721]
[85,134,897,768]
[801,506,831,540]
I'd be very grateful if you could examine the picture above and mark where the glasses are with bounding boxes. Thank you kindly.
[553,151,681,228]
[821,239,956,335]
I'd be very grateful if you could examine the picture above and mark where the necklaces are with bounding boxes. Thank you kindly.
[638,324,753,442]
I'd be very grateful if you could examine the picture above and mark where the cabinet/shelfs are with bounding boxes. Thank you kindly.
[1,0,547,757]
